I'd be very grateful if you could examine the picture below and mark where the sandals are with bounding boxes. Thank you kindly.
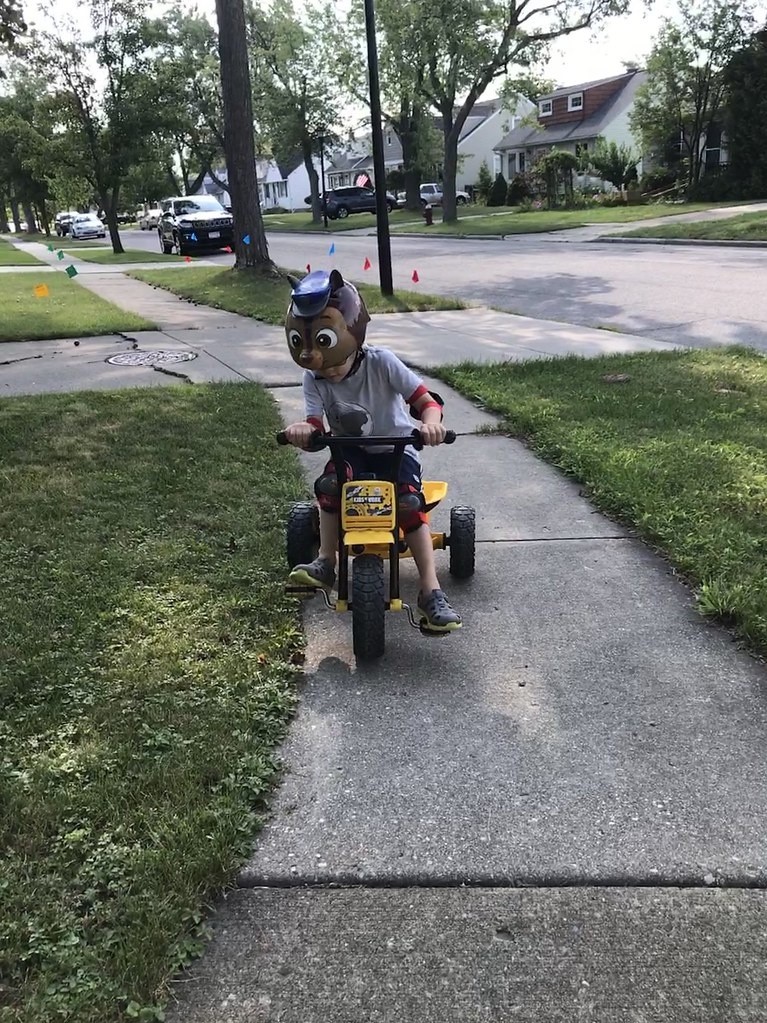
[288,556,338,595]
[416,587,462,631]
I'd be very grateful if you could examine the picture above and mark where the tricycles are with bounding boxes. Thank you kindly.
[276,430,478,662]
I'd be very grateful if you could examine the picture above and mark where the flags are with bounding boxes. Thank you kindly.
[48,244,53,252]
[57,250,64,260]
[329,244,335,255]
[243,235,250,244]
[65,265,78,278]
[364,257,371,269]
[412,269,419,283]
[34,283,49,298]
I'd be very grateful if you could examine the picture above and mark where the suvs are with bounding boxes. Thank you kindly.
[319,185,398,221]
[157,194,236,257]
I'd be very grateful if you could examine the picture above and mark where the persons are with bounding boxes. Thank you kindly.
[284,268,464,631]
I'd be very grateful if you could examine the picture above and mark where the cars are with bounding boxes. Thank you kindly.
[55,212,79,237]
[101,213,137,225]
[397,183,471,208]
[69,214,106,240]
[140,209,164,231]
[8,220,28,233]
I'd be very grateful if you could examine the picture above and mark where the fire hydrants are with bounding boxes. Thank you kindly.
[422,205,435,226]
